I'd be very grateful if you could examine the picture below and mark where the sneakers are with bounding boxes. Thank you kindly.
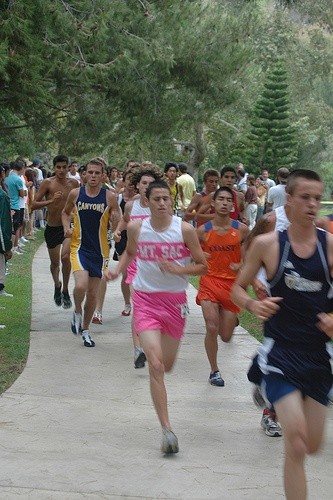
[81,329,96,347]
[91,311,102,325]
[71,309,82,336]
[134,349,147,368]
[122,304,131,316]
[161,431,179,454]
[54,281,62,306]
[261,408,282,437]
[253,385,267,410]
[208,371,224,387]
[62,291,72,309]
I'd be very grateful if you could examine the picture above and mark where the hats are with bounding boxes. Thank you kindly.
[247,175,256,184]
[278,168,290,183]
[22,157,33,166]
[33,159,41,164]
[178,162,188,172]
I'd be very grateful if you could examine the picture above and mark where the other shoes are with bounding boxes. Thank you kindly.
[19,237,28,242]
[18,240,25,248]
[0,325,6,329]
[11,247,23,255]
[0,290,13,297]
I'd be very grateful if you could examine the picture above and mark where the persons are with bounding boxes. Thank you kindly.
[197,187,250,387]
[100,180,207,454]
[241,205,333,436]
[231,169,333,500]
[0,156,333,369]
